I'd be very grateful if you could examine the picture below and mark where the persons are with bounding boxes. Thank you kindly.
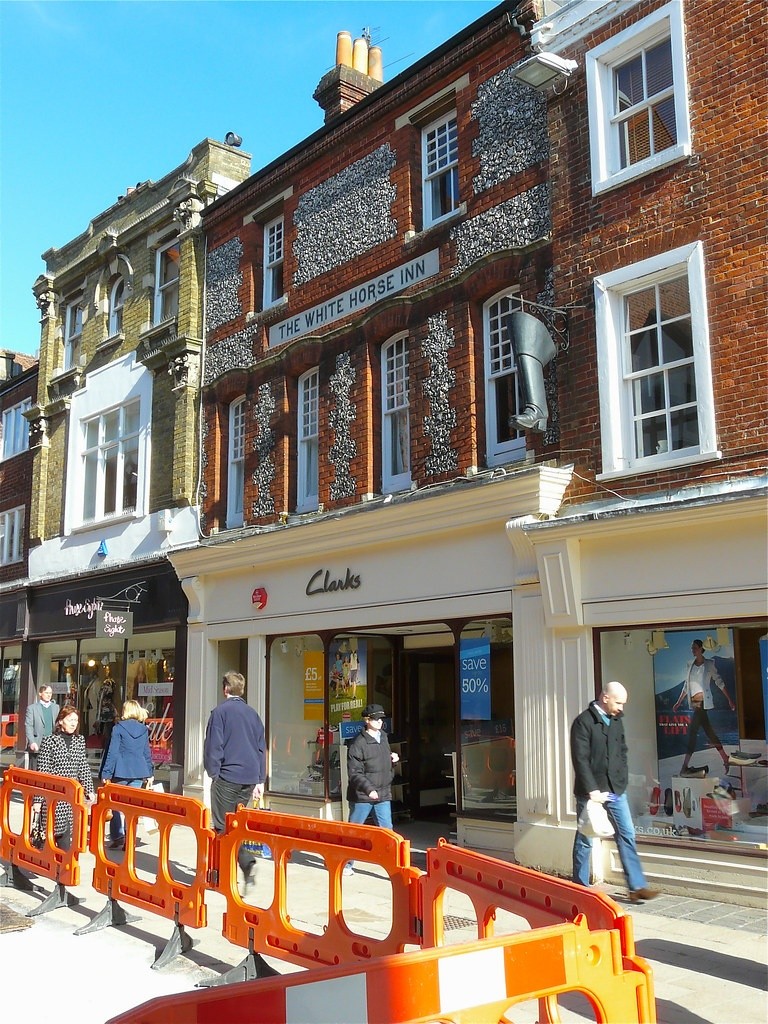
[98,699,154,851]
[24,684,60,771]
[96,666,119,735]
[204,673,266,896]
[673,640,736,776]
[329,649,360,700]
[84,662,103,736]
[64,666,75,706]
[163,658,174,718]
[134,662,153,707]
[31,705,95,853]
[343,704,399,876]
[570,682,658,903]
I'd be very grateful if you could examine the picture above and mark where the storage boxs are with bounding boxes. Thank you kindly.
[672,777,720,825]
[701,796,751,842]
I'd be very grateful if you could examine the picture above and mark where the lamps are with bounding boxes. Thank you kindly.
[224,132,243,147]
[145,650,152,660]
[64,658,70,667]
[81,654,88,663]
[156,650,164,659]
[133,651,139,661]
[510,53,579,91]
[108,652,117,662]
[71,656,76,665]
[281,640,288,653]
[101,655,108,666]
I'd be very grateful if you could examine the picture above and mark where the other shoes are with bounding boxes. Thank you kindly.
[728,750,768,766]
[723,756,731,775]
[630,887,660,902]
[18,867,38,881]
[342,867,354,876]
[683,787,692,819]
[243,861,258,897]
[649,787,661,815]
[675,791,682,812]
[680,765,709,778]
[109,836,127,851]
[664,788,673,816]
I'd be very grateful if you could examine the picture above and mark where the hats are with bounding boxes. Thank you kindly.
[361,704,386,721]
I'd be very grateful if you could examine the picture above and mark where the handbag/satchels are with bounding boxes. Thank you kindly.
[98,779,113,822]
[142,778,165,833]
[577,791,616,839]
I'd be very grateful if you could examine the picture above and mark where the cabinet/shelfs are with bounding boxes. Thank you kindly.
[340,741,410,824]
[443,751,459,844]
